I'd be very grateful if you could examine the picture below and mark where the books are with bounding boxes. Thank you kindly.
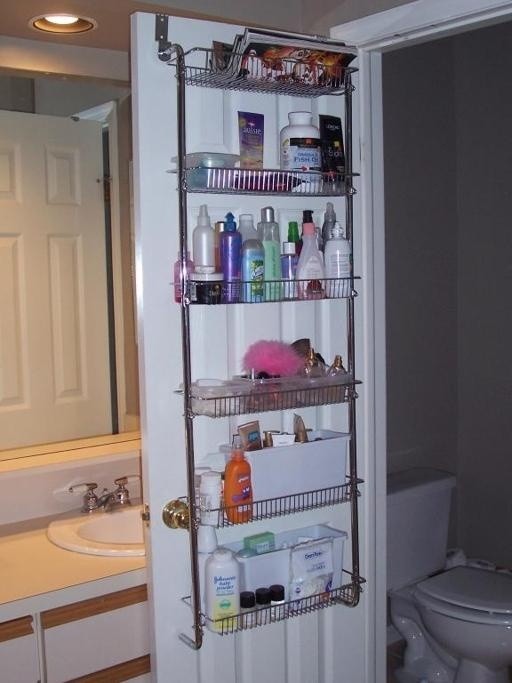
[207,27,356,87]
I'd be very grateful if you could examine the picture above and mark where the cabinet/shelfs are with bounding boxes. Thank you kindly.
[1,582,150,680]
[152,12,368,652]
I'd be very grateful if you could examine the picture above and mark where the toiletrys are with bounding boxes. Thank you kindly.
[193,434,287,631]
[174,200,351,303]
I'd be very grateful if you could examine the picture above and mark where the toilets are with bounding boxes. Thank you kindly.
[386,467,512,683]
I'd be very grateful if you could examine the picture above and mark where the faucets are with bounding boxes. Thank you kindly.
[97,492,116,512]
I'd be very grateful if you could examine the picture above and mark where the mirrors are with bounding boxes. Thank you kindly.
[1,37,142,461]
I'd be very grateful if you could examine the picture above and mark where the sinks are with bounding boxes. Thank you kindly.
[46,498,146,556]
